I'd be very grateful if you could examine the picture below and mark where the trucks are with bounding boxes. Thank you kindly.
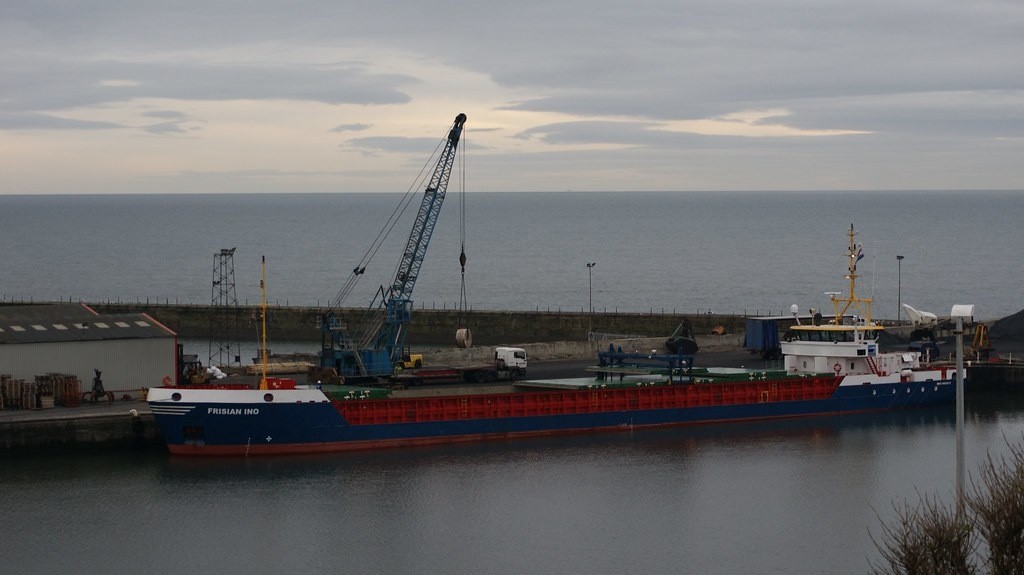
[711,325,727,336]
[459,347,529,383]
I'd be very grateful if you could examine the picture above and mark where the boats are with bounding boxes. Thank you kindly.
[145,220,972,462]
[667,316,700,356]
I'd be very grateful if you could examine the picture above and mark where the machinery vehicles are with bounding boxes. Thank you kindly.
[968,321,994,360]
[315,111,471,386]
[389,347,425,370]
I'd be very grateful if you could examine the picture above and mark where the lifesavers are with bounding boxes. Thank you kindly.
[163,375,171,385]
[834,364,842,372]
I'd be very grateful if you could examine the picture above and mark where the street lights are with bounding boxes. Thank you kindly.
[896,253,907,325]
[950,300,978,526]
[584,259,596,345]
